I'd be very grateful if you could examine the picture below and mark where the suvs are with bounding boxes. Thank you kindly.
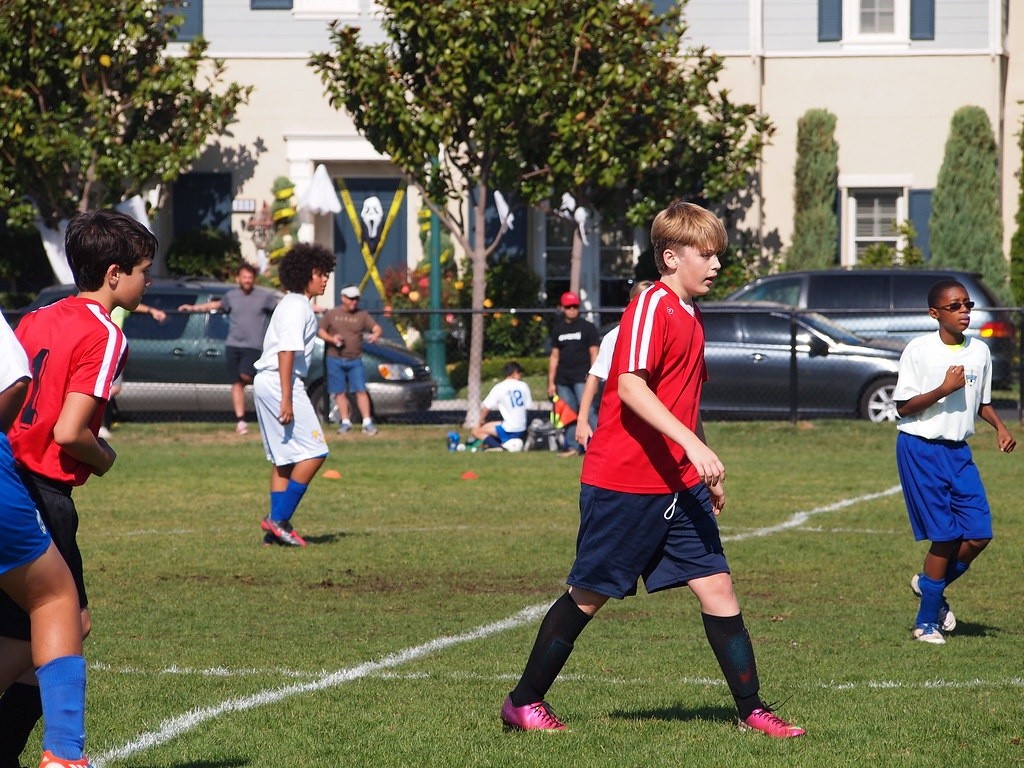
[719,264,1016,392]
[23,275,437,426]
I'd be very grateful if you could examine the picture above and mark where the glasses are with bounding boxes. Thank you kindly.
[933,302,974,310]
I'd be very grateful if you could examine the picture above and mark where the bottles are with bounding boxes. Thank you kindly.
[446,432,460,452]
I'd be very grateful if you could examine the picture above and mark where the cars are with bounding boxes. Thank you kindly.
[591,300,907,425]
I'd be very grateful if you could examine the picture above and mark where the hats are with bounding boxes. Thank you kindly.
[341,286,361,298]
[561,292,580,307]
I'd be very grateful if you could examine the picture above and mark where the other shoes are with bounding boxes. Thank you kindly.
[39,750,93,768]
[557,446,578,457]
[336,420,352,434]
[361,423,378,435]
[236,421,248,435]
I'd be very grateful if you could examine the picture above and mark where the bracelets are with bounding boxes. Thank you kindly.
[148,306,152,313]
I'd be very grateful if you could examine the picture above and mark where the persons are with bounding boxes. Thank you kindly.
[0,206,168,768]
[499,202,805,741]
[575,279,658,453]
[316,283,382,436]
[891,280,1017,644]
[546,292,600,458]
[179,264,325,436]
[251,242,339,547]
[470,362,533,453]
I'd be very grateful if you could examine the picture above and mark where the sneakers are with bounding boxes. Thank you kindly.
[738,695,806,739]
[912,623,946,645]
[911,573,956,632]
[262,513,305,546]
[263,532,288,545]
[500,692,566,733]
